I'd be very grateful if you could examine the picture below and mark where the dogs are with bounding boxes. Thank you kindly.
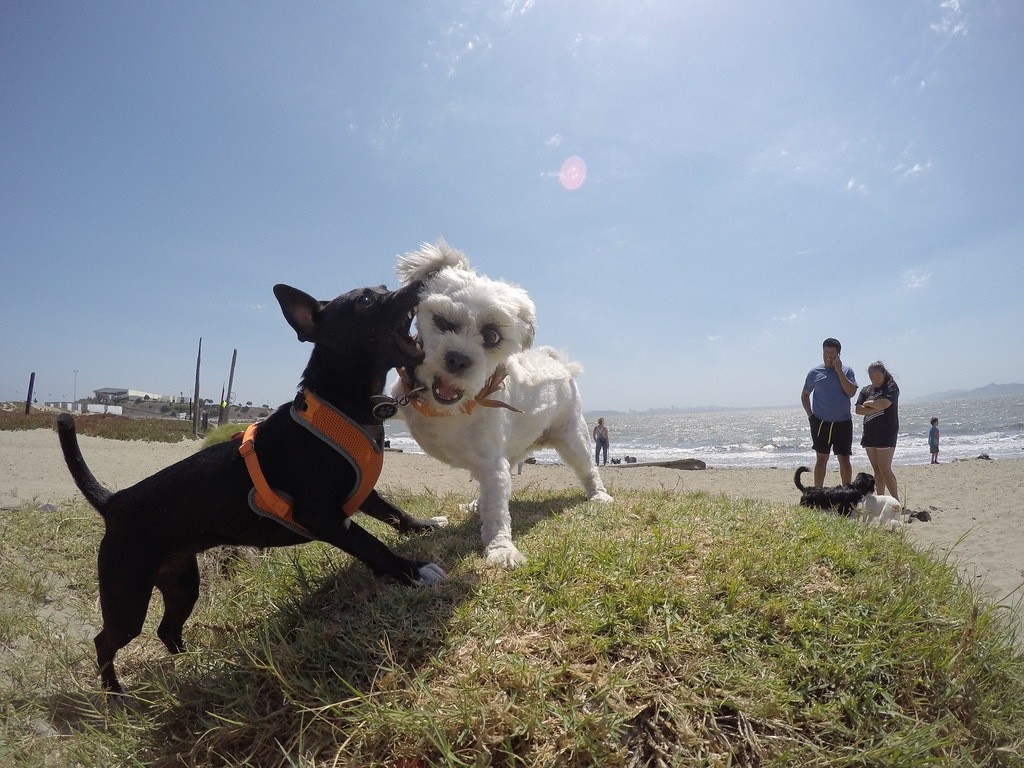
[57,234,615,701]
[793,466,903,531]
[610,455,637,465]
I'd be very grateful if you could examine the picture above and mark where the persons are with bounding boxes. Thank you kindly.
[928,417,940,465]
[855,360,900,503]
[593,418,609,465]
[801,338,858,488]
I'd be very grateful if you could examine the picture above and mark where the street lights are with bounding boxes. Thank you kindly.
[73,369,80,411]
